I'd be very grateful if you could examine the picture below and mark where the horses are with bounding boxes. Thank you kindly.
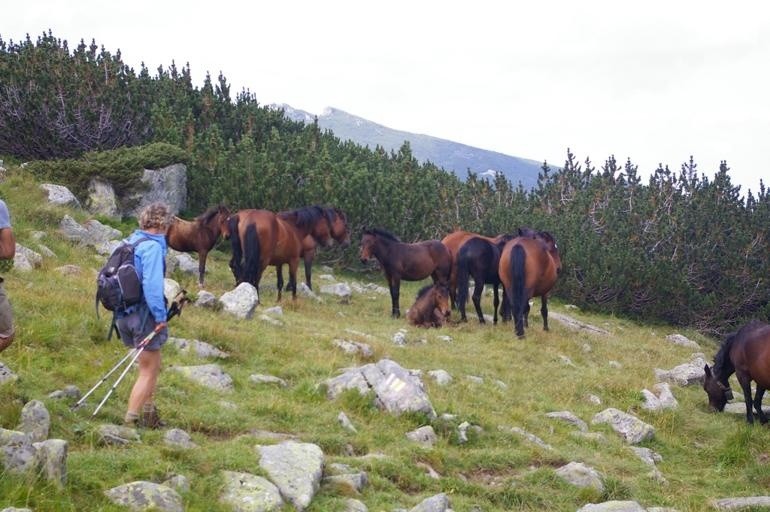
[438,230,503,311]
[403,278,455,331]
[496,231,561,341]
[240,204,335,306]
[702,320,770,428]
[455,227,526,326]
[229,204,351,291]
[356,225,455,321]
[166,204,234,289]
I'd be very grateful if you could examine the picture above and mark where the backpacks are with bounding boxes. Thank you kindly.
[95,233,168,312]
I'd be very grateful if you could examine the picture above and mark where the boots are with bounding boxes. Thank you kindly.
[123,410,167,428]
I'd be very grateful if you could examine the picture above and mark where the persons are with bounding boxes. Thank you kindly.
[96,202,173,432]
[1,198,15,353]
